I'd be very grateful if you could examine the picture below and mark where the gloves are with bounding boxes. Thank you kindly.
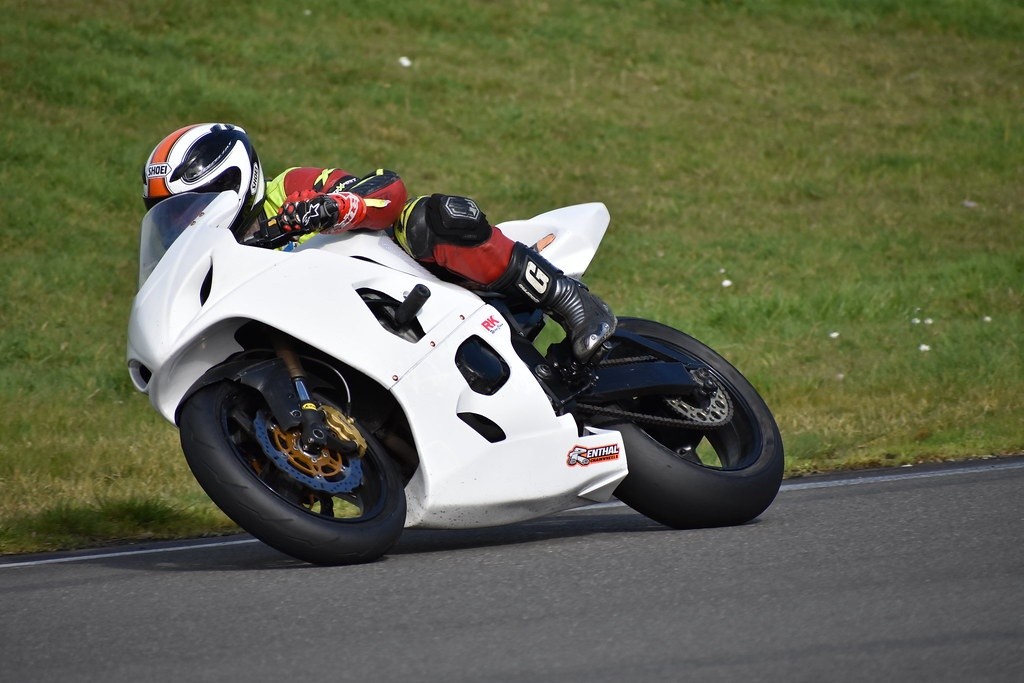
[278,189,340,233]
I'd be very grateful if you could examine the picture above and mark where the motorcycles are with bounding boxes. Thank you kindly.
[122,191,789,565]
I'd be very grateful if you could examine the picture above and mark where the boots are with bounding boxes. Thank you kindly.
[487,241,618,362]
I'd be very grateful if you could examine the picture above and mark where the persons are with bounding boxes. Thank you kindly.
[144,123,618,368]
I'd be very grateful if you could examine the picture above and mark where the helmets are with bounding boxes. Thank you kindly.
[142,123,264,250]
